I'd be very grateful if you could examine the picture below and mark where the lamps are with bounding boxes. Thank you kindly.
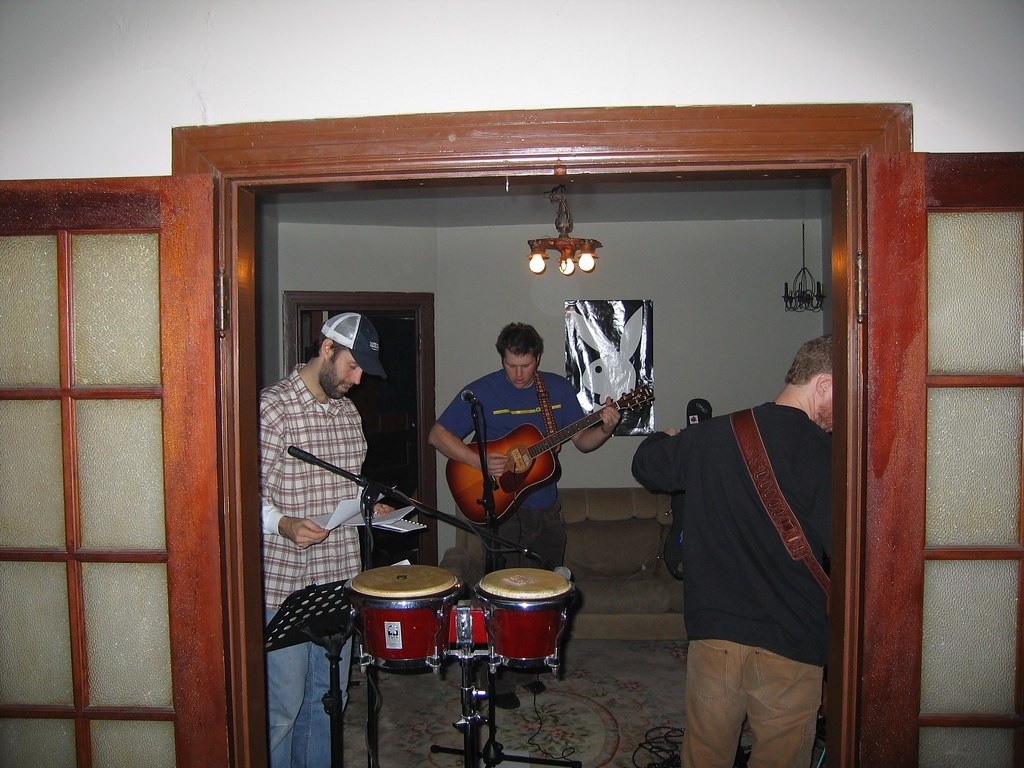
[782,223,824,312]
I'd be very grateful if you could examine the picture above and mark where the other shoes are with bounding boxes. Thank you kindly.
[522,680,546,694]
[494,693,520,710]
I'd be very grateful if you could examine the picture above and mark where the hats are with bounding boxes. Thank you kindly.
[321,312,386,377]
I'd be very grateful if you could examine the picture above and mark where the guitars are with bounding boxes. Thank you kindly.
[446,379,656,524]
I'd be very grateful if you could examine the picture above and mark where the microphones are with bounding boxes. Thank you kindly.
[525,551,571,582]
[461,390,479,406]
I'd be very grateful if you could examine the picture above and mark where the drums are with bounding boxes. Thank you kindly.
[351,564,462,669]
[474,568,576,669]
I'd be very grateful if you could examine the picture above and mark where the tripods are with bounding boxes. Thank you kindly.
[431,405,583,768]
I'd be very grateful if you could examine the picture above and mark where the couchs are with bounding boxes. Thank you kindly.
[439,487,688,639]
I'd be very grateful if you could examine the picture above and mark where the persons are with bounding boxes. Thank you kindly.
[427,323,620,710]
[258,312,395,768]
[632,334,834,768]
